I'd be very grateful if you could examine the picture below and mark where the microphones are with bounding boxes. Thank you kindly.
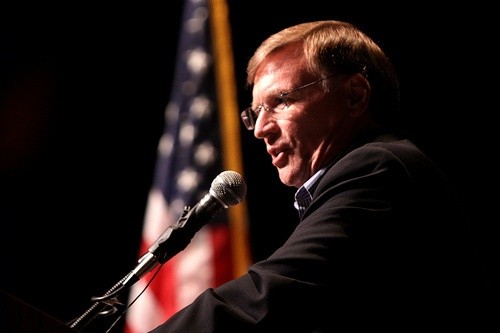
[121,170,247,288]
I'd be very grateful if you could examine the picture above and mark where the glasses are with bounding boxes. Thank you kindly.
[241,74,341,131]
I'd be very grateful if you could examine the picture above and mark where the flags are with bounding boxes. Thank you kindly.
[124,0,237,333]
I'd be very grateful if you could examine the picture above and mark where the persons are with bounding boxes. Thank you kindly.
[148,19,500,333]
